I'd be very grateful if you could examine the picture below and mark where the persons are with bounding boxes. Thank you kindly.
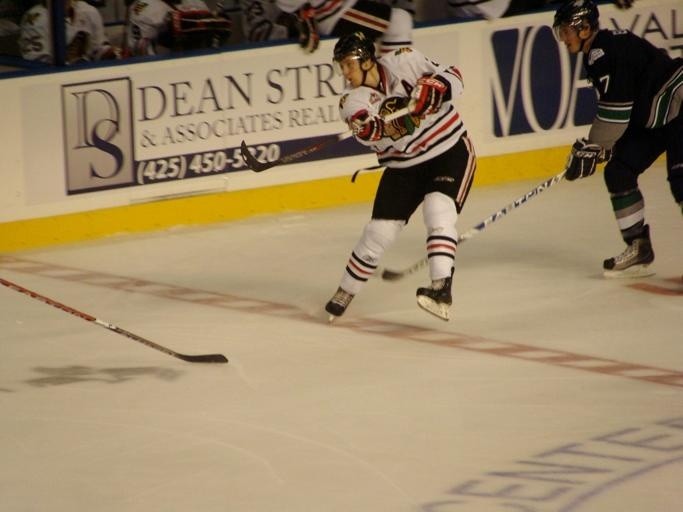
[552,0,683,270]
[325,31,476,317]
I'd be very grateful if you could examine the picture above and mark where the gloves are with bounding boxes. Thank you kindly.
[406,74,447,121]
[596,146,613,163]
[562,138,598,182]
[347,109,385,143]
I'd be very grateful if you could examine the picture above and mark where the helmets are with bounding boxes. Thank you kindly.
[331,29,376,64]
[551,0,600,29]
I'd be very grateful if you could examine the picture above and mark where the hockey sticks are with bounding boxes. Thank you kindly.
[240,103,415,173]
[2,279,228,363]
[382,162,566,282]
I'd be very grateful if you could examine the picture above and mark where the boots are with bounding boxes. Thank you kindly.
[414,264,458,307]
[324,285,356,317]
[600,224,656,273]
